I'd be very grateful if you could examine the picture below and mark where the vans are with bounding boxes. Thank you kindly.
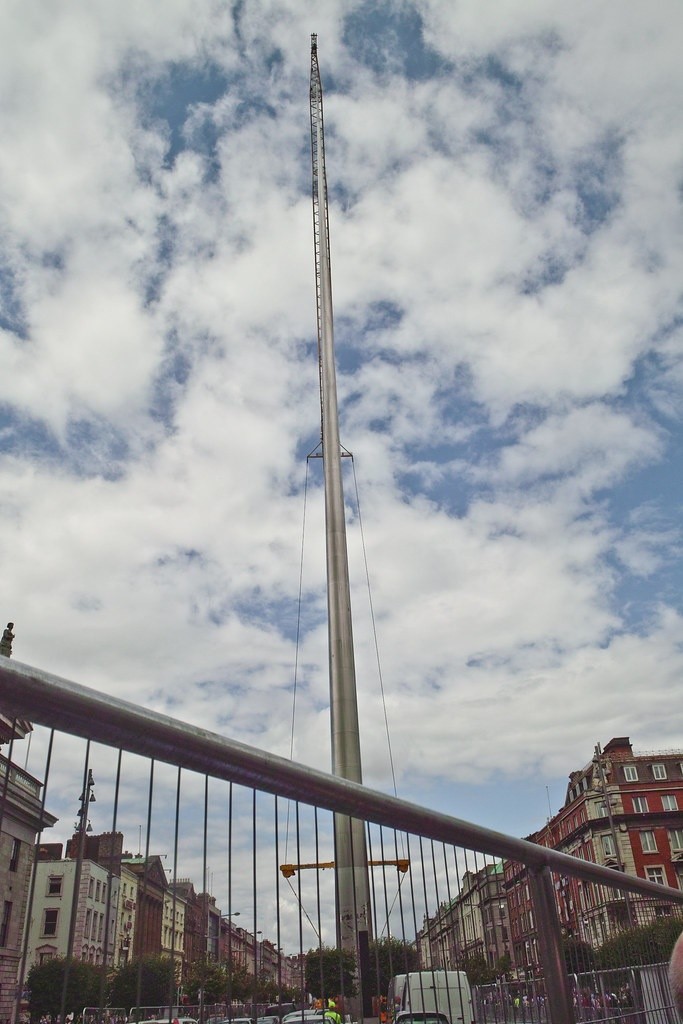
[385,974,408,1024]
[401,971,474,1024]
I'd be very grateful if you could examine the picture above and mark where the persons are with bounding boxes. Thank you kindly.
[379,986,634,1024]
[40,1008,208,1024]
[324,1001,343,1024]
[0,622,15,658]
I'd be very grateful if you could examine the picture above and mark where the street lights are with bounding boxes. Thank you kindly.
[464,903,498,982]
[216,912,240,963]
[245,930,262,968]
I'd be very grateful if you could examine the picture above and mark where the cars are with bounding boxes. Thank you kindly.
[391,1011,452,1024]
[129,1008,338,1024]
